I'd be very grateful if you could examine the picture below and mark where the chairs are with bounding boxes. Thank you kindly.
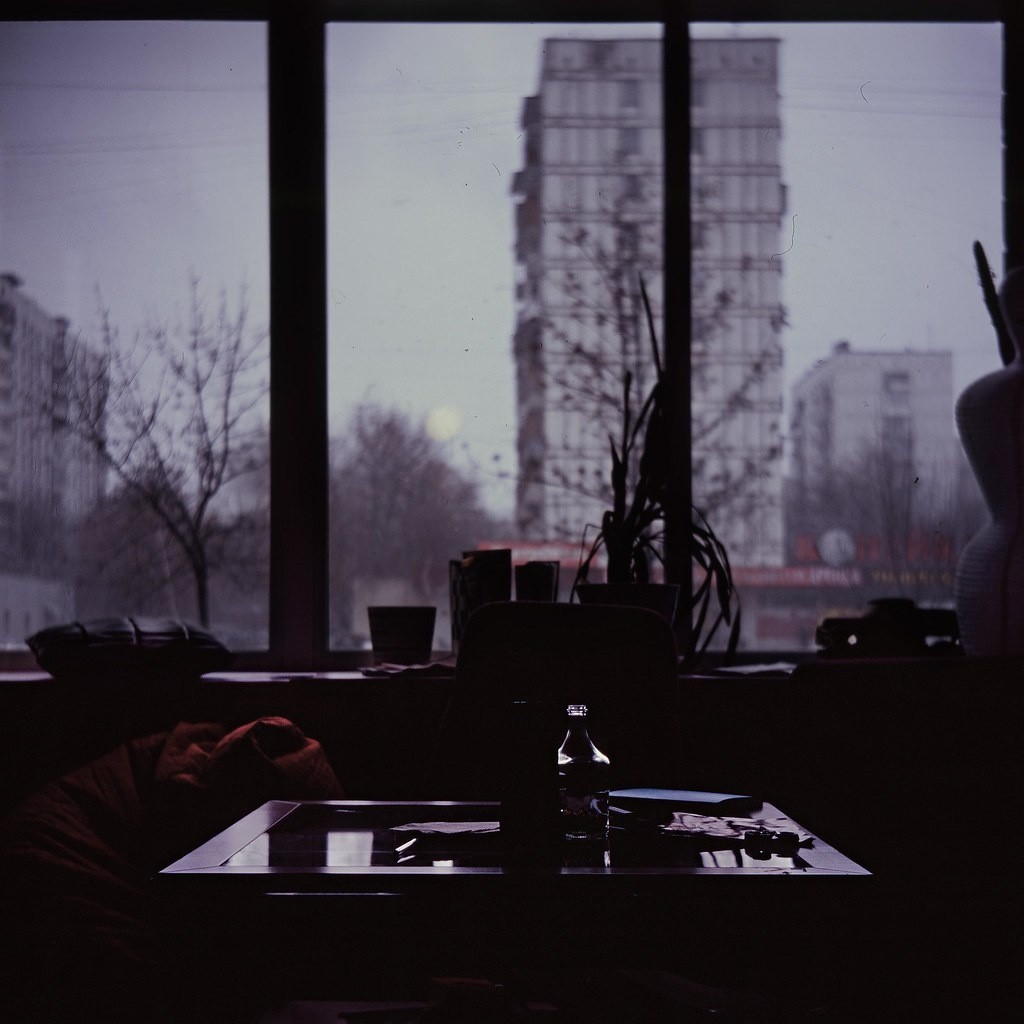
[439,600,676,789]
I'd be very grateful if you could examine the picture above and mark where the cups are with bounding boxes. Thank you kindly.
[369,607,435,663]
[515,561,559,603]
[449,549,511,656]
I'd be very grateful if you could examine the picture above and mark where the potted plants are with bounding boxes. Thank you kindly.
[571,273,743,659]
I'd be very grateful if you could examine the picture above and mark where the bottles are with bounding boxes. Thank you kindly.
[557,704,608,839]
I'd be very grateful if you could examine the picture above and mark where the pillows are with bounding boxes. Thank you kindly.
[24,618,239,680]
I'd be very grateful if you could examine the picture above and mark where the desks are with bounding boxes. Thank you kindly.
[160,797,872,1024]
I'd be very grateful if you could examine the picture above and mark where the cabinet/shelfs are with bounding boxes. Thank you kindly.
[1,654,1024,821]
